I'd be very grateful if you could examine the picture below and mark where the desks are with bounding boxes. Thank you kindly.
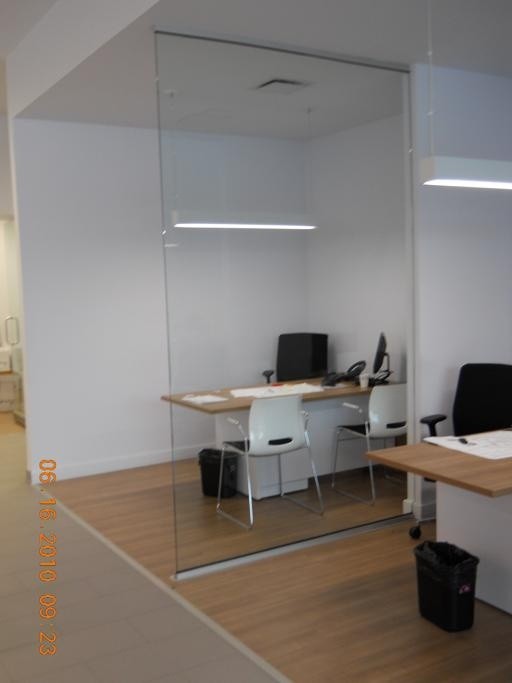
[365,427,512,616]
[160,375,397,501]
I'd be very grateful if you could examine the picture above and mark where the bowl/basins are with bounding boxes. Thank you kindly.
[0,344,11,375]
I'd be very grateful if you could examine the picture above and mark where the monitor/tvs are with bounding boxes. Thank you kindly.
[277,333,328,381]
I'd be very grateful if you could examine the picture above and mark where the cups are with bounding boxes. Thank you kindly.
[357,372,372,389]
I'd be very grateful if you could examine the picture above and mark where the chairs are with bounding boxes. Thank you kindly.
[216,393,324,530]
[419,363,512,482]
[262,333,328,383]
[330,384,408,506]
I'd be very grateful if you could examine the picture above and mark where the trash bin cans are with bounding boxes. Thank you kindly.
[198,448,240,499]
[413,540,481,633]
